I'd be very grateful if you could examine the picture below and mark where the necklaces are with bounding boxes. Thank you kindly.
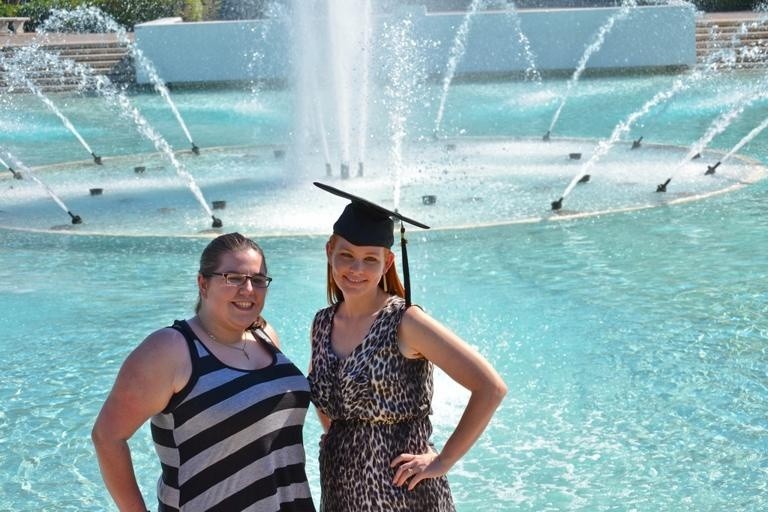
[192,314,253,362]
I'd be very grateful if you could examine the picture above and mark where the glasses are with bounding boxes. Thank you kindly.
[211,271,274,289]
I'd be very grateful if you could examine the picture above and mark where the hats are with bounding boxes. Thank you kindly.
[312,181,430,310]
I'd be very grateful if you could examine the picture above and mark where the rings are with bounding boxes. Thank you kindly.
[403,466,416,477]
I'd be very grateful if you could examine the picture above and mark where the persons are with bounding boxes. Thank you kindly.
[89,231,317,511]
[307,181,507,511]
